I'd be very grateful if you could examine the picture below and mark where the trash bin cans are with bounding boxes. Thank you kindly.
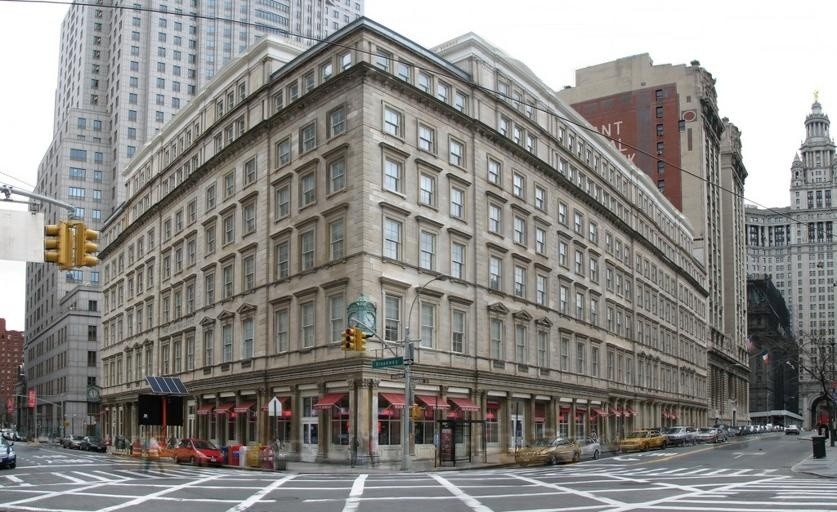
[277,452,288,469]
[813,436,826,458]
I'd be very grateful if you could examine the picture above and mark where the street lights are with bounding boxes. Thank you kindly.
[717,361,740,424]
[401,274,451,469]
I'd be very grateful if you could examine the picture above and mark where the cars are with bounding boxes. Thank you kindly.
[617,422,801,455]
[173,438,226,467]
[58,432,106,453]
[0,428,27,469]
[513,436,601,467]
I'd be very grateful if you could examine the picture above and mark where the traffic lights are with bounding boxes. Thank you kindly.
[346,327,356,350]
[44,222,74,270]
[357,328,366,351]
[75,222,97,267]
[341,330,346,349]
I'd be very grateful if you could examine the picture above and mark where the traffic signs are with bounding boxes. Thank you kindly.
[373,357,403,369]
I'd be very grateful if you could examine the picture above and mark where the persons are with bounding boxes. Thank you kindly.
[142,436,166,473]
